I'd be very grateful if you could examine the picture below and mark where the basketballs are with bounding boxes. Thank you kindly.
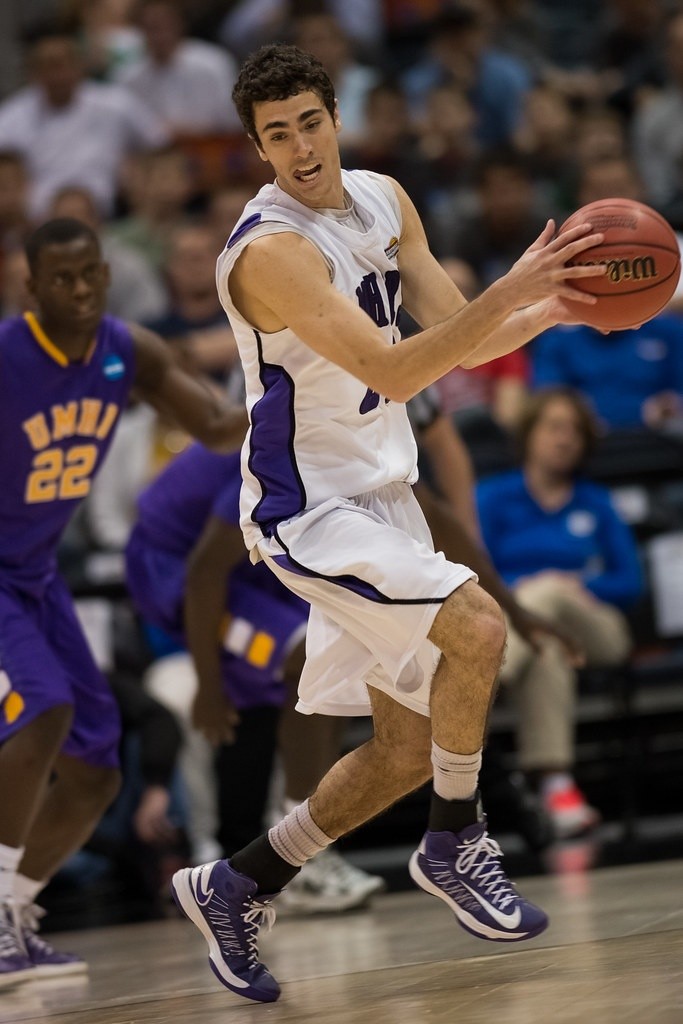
[558,195,682,331]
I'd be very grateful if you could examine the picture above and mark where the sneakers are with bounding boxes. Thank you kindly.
[0,895,37,990]
[172,857,281,1000]
[281,848,385,910]
[16,902,88,978]
[409,819,549,943]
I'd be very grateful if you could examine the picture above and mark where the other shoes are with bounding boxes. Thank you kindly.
[543,784,598,838]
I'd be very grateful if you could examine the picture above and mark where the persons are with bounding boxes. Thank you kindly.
[1,1,683,911]
[1,217,249,989]
[171,42,607,1000]
